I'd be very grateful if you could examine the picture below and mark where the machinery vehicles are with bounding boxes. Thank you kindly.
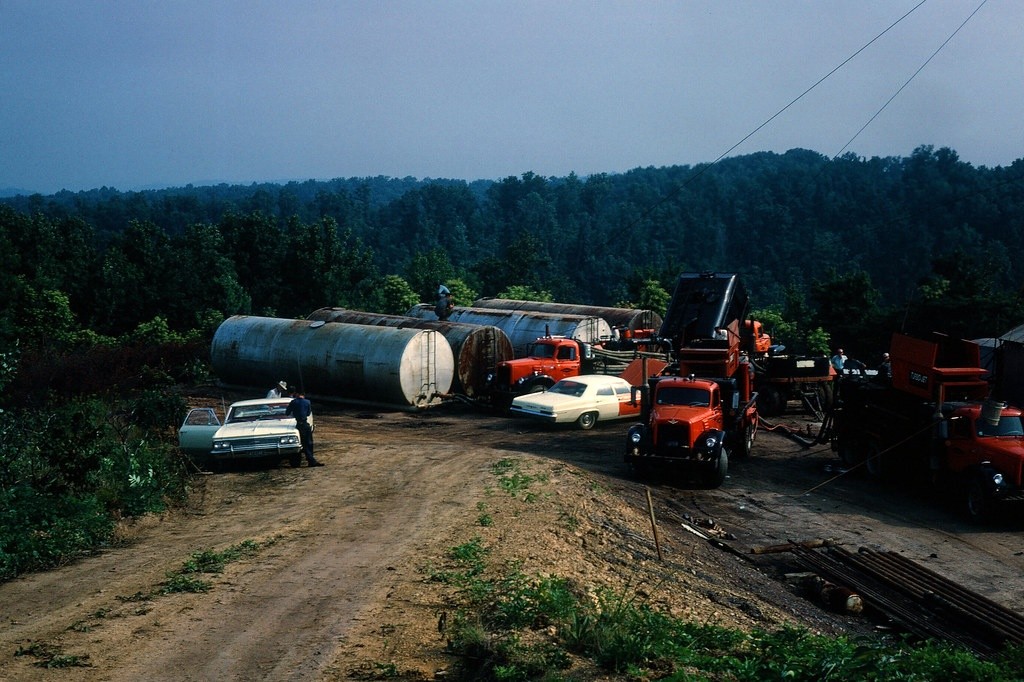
[480,269,1024,526]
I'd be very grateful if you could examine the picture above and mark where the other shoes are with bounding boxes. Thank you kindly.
[308,461,325,466]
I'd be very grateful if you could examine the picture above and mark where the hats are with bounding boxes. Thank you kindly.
[279,380,288,391]
[883,352,889,357]
[838,349,843,353]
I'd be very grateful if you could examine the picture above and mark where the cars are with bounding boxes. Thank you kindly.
[511,373,645,429]
[179,391,316,472]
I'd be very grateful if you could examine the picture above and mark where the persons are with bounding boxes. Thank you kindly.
[286,392,325,467]
[266,381,287,398]
[434,280,450,301]
[611,326,620,341]
[832,349,848,374]
[881,353,892,375]
[435,293,454,321]
